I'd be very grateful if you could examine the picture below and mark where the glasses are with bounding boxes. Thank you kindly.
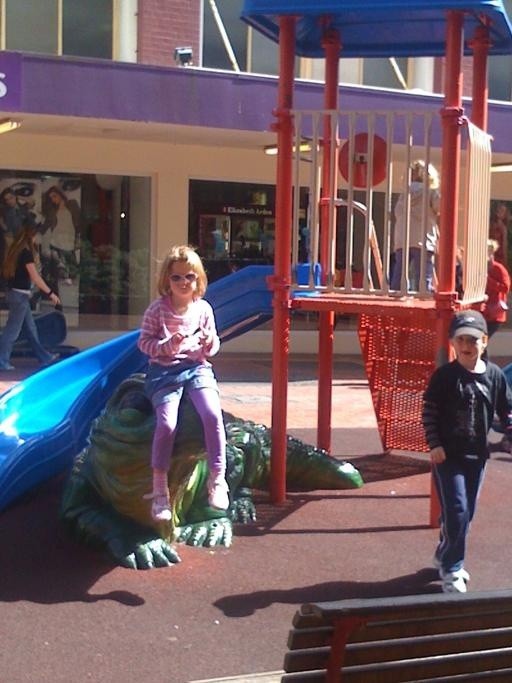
[167,273,198,282]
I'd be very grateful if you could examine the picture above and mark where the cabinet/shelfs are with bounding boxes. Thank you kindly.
[194,203,308,263]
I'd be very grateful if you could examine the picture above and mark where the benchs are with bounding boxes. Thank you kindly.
[185,589,511,683]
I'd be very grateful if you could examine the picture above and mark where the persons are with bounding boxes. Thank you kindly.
[451,243,464,299]
[136,244,231,524]
[1,220,62,369]
[388,157,441,295]
[419,309,510,593]
[489,202,511,269]
[2,185,36,286]
[465,236,511,363]
[38,184,81,288]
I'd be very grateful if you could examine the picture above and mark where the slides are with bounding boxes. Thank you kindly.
[0,263,321,507]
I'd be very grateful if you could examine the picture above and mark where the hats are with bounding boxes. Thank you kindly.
[449,309,489,337]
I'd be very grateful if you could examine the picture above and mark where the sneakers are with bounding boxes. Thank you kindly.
[206,477,231,510]
[143,490,171,521]
[432,557,470,594]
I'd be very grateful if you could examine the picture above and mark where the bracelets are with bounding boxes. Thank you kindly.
[49,289,54,296]
